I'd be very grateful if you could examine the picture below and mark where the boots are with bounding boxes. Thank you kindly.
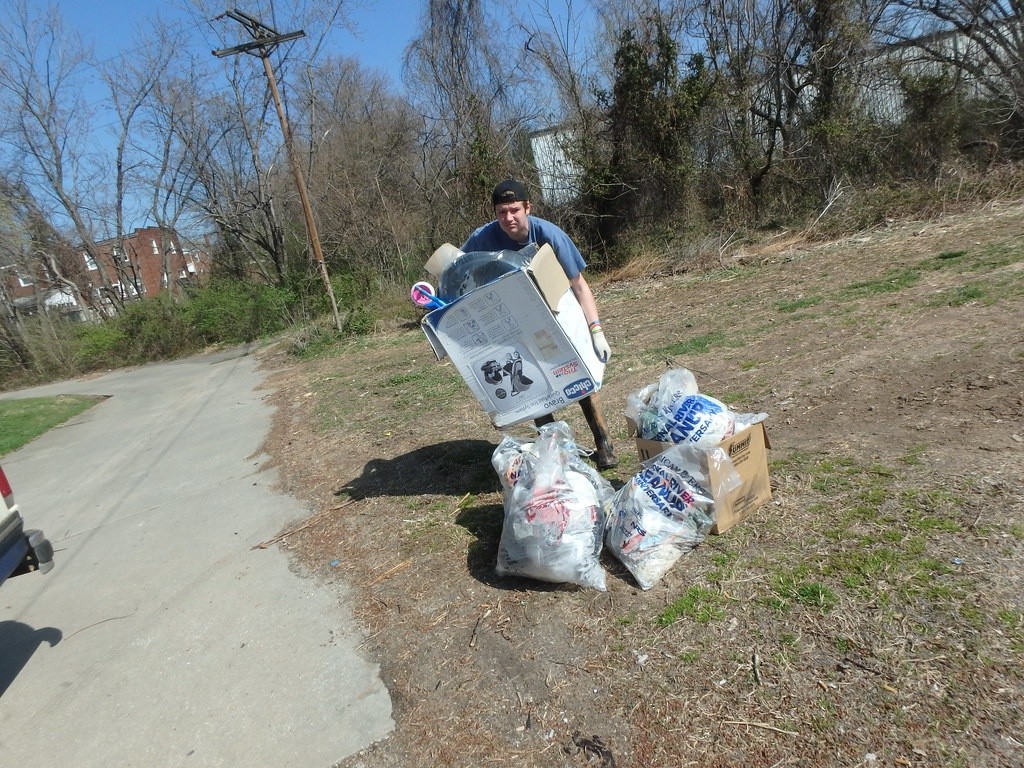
[534,415,555,435]
[580,393,618,467]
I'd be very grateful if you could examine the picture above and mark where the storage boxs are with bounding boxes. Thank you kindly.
[420,243,606,429]
[626,411,773,533]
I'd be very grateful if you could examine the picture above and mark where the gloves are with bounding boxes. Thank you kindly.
[592,325,611,363]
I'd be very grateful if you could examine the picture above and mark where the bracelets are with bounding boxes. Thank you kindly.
[589,323,603,330]
[591,329,604,334]
[589,320,601,328]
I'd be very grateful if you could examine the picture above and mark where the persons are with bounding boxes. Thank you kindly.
[437,179,621,471]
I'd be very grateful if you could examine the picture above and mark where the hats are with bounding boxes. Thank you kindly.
[493,180,528,205]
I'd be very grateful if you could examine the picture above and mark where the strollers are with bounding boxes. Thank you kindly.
[501,351,533,396]
[411,251,530,311]
